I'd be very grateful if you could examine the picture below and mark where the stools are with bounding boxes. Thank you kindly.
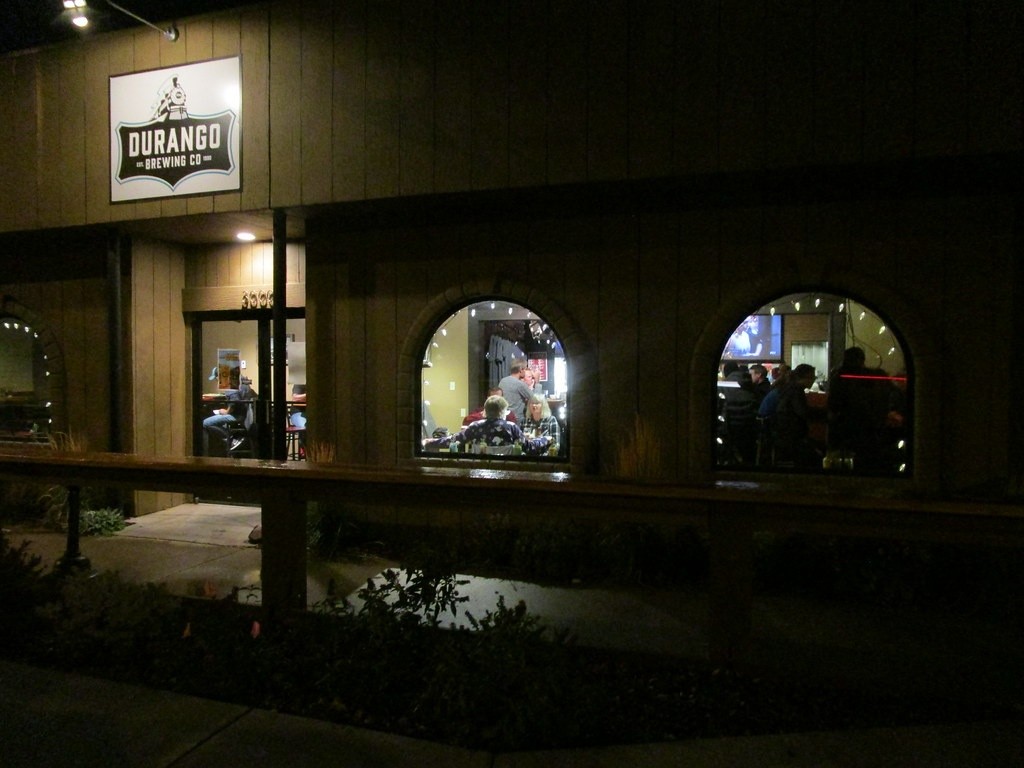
[282,425,306,462]
[225,418,254,459]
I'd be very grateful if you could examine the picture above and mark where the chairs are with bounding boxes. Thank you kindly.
[719,402,755,469]
[754,413,795,469]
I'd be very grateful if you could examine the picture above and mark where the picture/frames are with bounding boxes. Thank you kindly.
[528,352,548,381]
[270,333,295,366]
[106,54,244,205]
[216,349,241,392]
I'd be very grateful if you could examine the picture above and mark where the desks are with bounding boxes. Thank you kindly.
[545,397,566,436]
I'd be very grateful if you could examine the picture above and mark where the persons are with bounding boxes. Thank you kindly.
[517,394,560,455]
[289,384,309,430]
[523,368,541,392]
[421,395,554,455]
[202,367,251,450]
[462,386,517,424]
[499,358,543,423]
[719,348,912,480]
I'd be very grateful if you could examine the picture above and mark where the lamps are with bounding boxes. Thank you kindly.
[60,0,90,31]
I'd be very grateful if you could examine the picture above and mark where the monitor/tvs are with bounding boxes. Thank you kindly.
[719,313,785,364]
